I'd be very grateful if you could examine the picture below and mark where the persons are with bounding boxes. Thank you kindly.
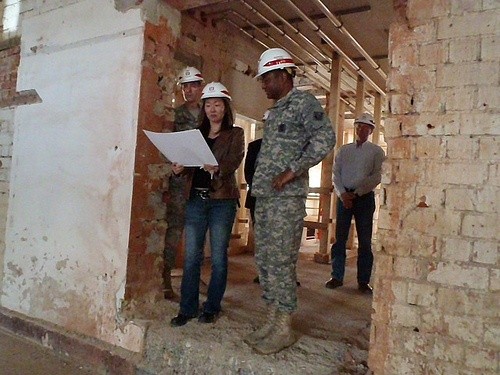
[162,66,206,302]
[247,110,301,287]
[325,112,386,295]
[169,81,246,325]
[244,48,336,353]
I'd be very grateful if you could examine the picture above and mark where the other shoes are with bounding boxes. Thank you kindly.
[170,313,196,326]
[199,311,218,322]
[163,291,181,303]
[254,276,259,283]
[325,277,342,288]
[358,283,372,293]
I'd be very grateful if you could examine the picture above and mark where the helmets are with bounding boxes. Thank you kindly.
[178,66,203,83]
[353,113,375,129]
[200,81,232,101]
[253,48,295,79]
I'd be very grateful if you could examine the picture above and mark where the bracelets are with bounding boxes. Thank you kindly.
[353,191,359,197]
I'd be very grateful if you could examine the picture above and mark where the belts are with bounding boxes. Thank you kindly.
[191,189,208,199]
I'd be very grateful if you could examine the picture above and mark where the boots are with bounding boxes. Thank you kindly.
[252,310,296,354]
[243,306,278,345]
